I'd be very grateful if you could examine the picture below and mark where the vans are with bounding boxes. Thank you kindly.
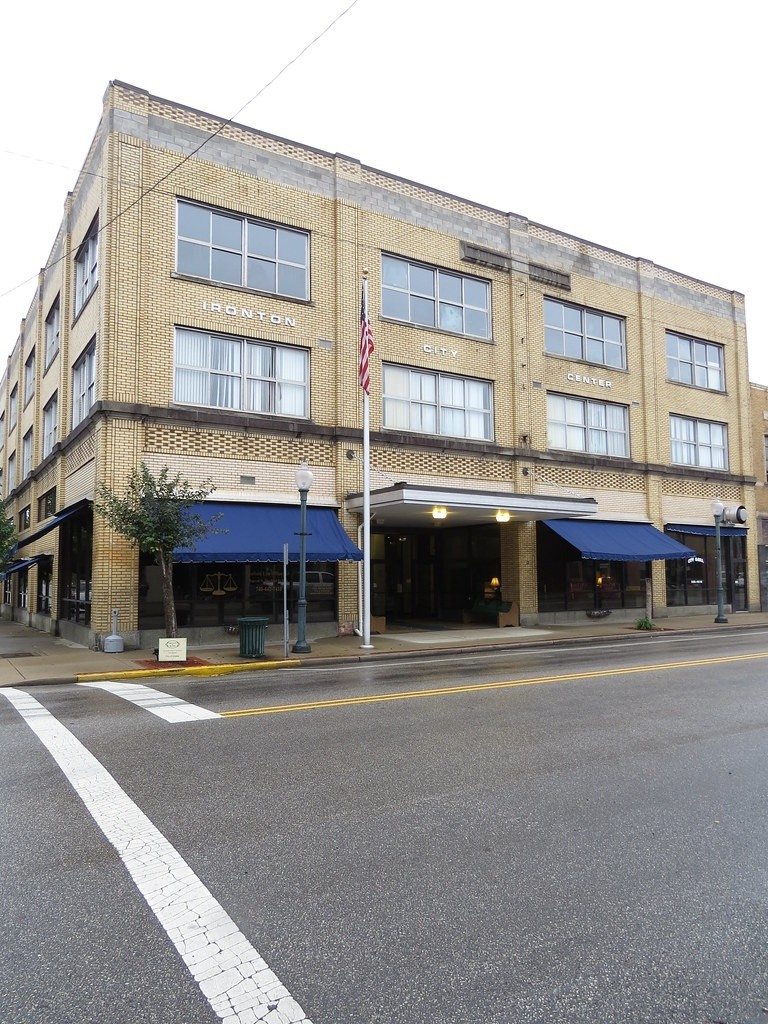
[67,581,93,612]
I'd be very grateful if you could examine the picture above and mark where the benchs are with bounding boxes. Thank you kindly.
[462,601,520,628]
[370,614,386,634]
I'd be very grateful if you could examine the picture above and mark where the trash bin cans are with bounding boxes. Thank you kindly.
[237,617,270,659]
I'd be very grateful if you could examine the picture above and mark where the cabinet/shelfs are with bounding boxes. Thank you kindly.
[482,581,501,600]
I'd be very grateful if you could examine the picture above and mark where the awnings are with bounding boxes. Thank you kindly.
[665,521,749,536]
[542,520,696,560]
[0,505,90,584]
[147,500,364,563]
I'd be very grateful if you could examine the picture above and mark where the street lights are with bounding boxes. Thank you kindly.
[709,493,729,621]
[295,457,316,654]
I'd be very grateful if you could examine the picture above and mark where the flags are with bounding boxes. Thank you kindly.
[358,279,376,395]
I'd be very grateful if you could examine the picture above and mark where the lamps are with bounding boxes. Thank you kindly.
[490,577,500,591]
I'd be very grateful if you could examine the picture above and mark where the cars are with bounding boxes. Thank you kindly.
[292,571,338,598]
